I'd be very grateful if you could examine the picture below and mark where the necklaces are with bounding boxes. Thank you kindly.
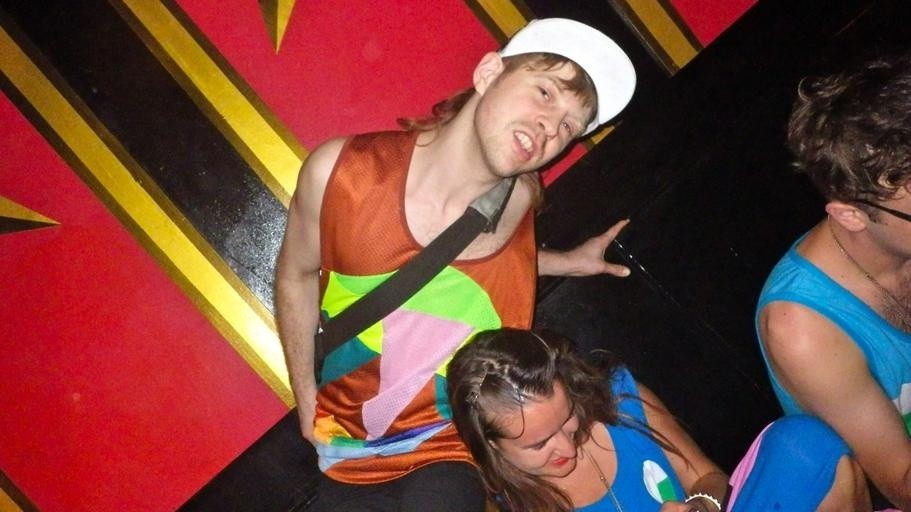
[581,445,623,512]
[827,213,911,315]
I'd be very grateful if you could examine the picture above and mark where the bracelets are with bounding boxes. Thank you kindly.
[684,492,723,512]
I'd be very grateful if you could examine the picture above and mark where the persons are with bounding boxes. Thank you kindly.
[271,13,637,512]
[446,325,877,512]
[755,54,911,512]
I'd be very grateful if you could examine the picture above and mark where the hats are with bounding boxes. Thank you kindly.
[496,17,637,137]
